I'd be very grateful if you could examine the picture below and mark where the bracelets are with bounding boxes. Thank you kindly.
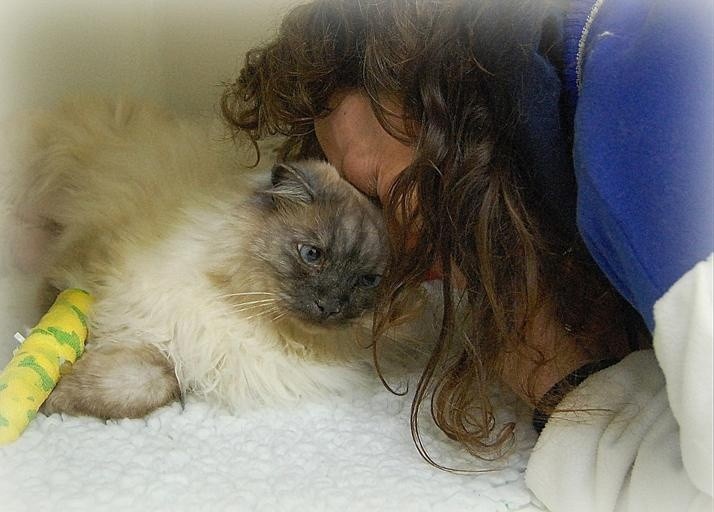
[531,362,597,433]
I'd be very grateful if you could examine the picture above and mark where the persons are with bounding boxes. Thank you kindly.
[217,0,714,512]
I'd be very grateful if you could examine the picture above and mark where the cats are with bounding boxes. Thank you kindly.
[0,77,472,421]
